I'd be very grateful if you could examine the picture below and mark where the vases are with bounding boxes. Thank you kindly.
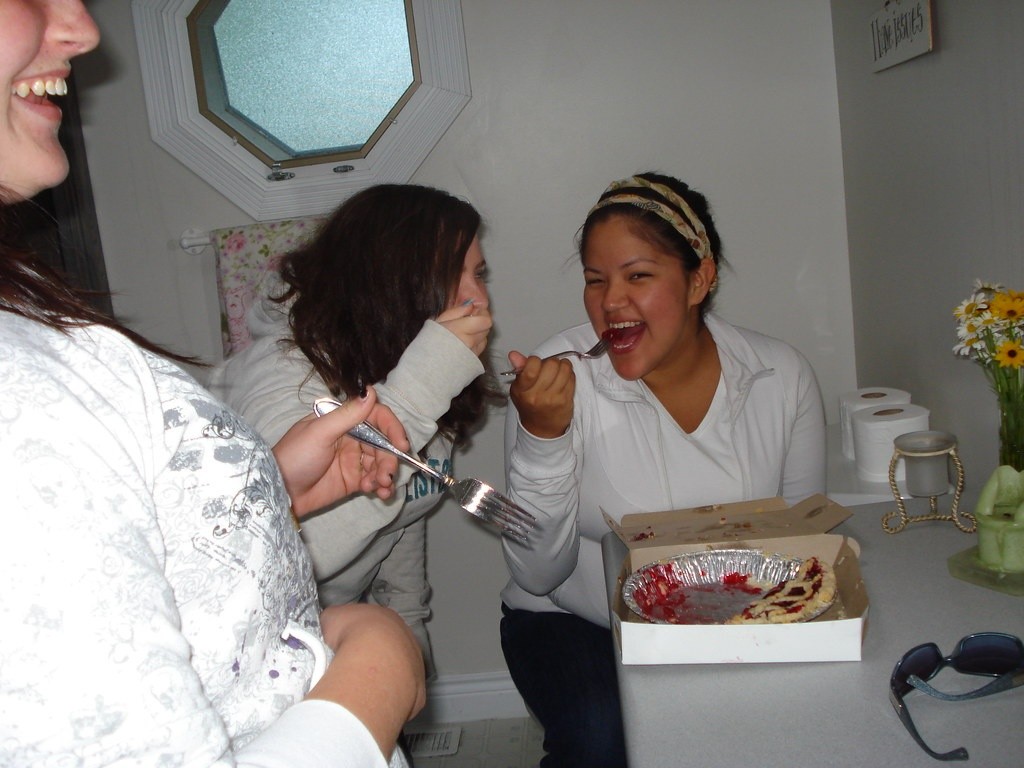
[946,464,1024,597]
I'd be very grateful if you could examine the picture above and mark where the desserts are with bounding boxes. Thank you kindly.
[724,556,837,625]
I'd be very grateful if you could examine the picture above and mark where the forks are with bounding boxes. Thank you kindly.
[314,397,539,542]
[500,328,623,376]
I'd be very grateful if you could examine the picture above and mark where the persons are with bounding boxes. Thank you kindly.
[494,170,832,767]
[2,0,433,768]
[200,177,499,690]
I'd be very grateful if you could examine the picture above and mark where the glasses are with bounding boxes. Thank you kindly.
[889,632,1024,762]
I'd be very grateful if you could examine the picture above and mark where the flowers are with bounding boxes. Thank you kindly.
[953,277,1024,472]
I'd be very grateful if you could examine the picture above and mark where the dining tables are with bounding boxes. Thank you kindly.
[601,492,1024,768]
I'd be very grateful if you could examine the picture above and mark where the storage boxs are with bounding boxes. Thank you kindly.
[599,495,869,665]
